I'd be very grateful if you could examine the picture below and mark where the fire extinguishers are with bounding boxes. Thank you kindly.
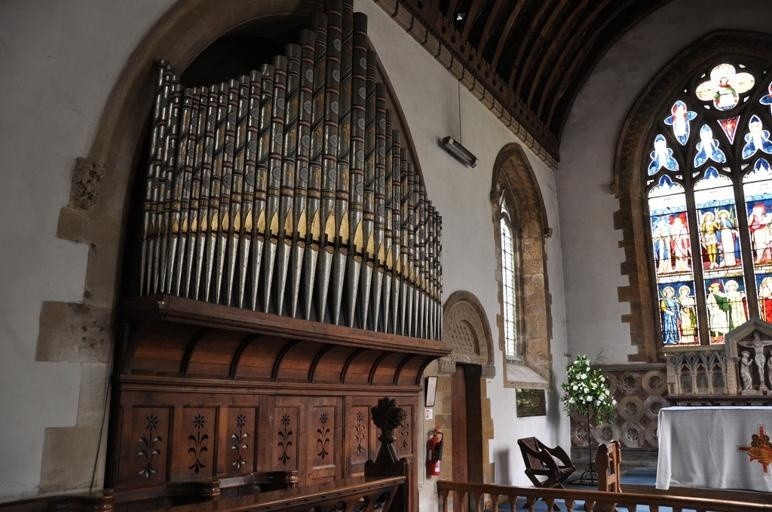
[428,427,444,476]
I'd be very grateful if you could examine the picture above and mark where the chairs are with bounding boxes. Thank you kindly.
[517,437,577,512]
[584,441,624,512]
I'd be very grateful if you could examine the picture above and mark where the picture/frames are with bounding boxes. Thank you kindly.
[425,376,438,407]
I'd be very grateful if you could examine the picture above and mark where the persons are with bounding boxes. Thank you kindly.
[739,351,753,391]
[651,200,771,345]
[766,349,772,390]
[739,340,770,389]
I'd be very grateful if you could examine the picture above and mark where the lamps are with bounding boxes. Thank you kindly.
[439,80,480,169]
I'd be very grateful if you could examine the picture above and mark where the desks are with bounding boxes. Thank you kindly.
[655,406,772,493]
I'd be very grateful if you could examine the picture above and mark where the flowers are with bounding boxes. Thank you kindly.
[559,351,617,428]
